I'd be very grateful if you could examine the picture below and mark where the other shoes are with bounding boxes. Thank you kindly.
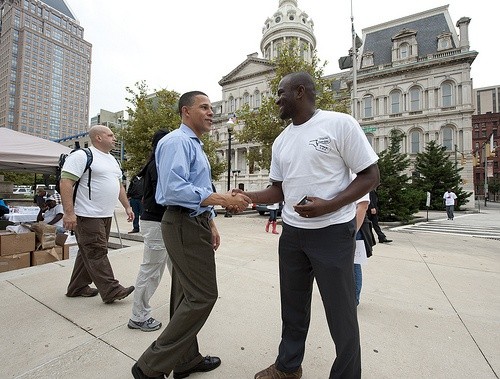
[379,238,392,243]
[254,364,302,379]
[128,230,139,233]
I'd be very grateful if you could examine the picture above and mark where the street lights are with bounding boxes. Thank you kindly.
[226,118,234,190]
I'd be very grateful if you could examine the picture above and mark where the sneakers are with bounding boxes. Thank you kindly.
[105,284,135,304]
[67,285,98,297]
[128,317,162,332]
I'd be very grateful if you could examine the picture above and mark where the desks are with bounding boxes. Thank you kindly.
[4,212,38,225]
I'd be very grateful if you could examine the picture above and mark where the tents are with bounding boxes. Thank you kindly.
[0,126,74,193]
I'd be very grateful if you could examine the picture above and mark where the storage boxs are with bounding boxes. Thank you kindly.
[0,221,70,273]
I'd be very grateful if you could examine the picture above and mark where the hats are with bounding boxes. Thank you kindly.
[45,197,56,202]
[37,187,44,193]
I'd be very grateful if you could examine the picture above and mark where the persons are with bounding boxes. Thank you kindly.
[34,188,45,206]
[38,197,65,233]
[127,167,144,234]
[60,125,135,304]
[354,192,370,304]
[128,129,172,331]
[367,189,393,243]
[0,205,16,230]
[132,91,252,379]
[225,70,380,379]
[265,202,279,234]
[443,188,458,220]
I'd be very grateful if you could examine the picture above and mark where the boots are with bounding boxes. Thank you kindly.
[272,221,279,234]
[265,218,273,232]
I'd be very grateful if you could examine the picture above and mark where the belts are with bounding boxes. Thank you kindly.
[167,205,211,217]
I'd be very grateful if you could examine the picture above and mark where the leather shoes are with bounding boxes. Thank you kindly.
[173,356,221,379]
[131,363,165,379]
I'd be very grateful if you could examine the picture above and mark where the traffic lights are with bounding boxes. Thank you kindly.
[473,154,480,167]
[461,156,467,165]
[485,144,495,158]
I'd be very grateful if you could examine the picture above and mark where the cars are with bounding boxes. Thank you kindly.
[12,183,57,197]
[213,200,285,217]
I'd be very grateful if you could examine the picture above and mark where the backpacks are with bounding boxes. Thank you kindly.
[55,148,93,195]
